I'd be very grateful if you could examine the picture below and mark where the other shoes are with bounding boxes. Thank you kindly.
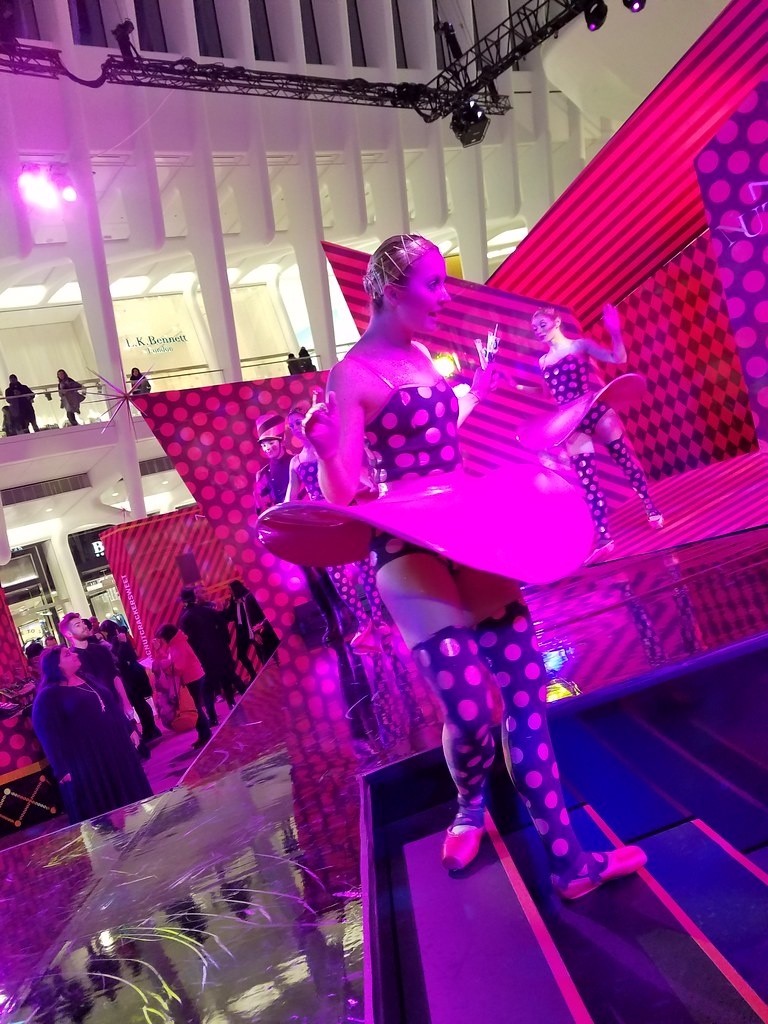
[442,821,487,871]
[649,514,664,529]
[322,624,340,643]
[550,846,648,900]
[193,733,213,747]
[588,539,616,563]
[208,718,220,727]
[142,727,164,743]
[229,702,236,711]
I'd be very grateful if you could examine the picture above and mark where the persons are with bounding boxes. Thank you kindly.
[252,415,351,643]
[286,354,304,375]
[193,581,281,710]
[32,644,155,826]
[151,624,212,749]
[298,347,312,373]
[504,303,664,566]
[130,368,151,395]
[2,374,40,437]
[57,369,86,426]
[25,640,44,658]
[255,234,648,900]
[285,401,392,648]
[45,612,162,745]
[177,587,236,726]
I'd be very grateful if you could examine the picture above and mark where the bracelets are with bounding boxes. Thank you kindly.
[469,390,481,402]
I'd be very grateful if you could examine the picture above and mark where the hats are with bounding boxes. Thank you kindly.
[256,414,286,443]
[178,588,197,602]
